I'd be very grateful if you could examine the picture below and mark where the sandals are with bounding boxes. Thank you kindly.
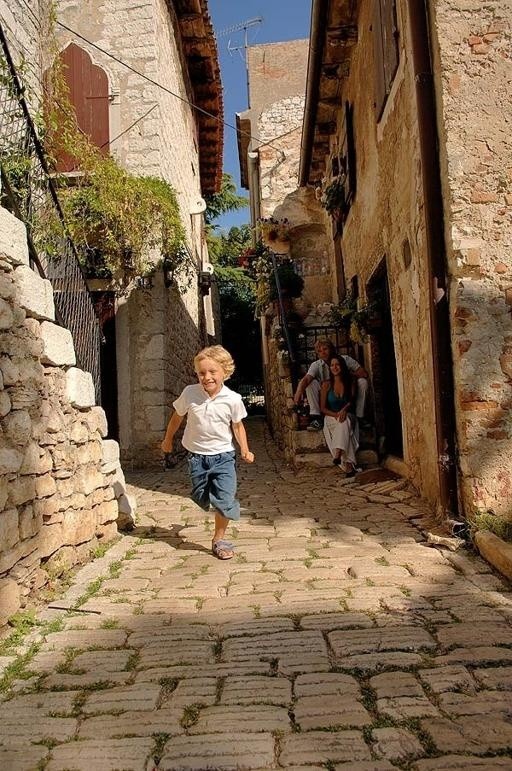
[212,538,235,561]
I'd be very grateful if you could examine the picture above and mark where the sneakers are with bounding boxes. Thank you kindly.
[307,420,323,431]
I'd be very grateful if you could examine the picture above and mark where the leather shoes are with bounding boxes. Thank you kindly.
[346,471,356,478]
[332,457,341,465]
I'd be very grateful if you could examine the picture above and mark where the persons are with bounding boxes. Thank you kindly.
[320,354,359,477]
[161,343,255,559]
[293,339,374,433]
